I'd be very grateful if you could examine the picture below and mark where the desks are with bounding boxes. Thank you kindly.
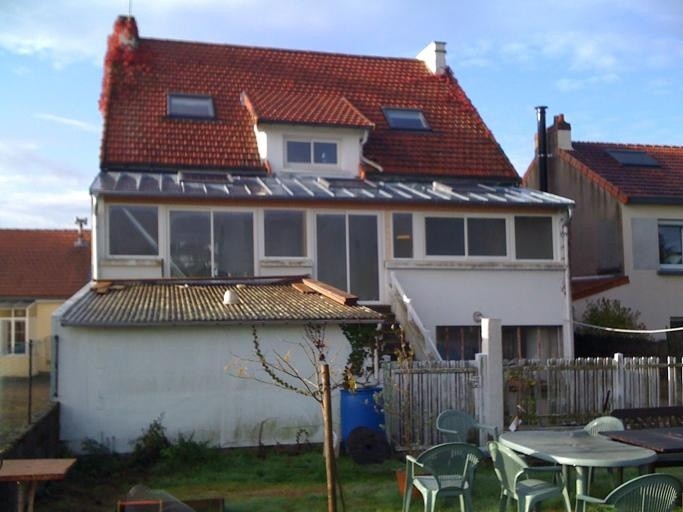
[0,458,78,512]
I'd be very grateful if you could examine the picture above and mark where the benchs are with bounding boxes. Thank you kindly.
[606,406,683,485]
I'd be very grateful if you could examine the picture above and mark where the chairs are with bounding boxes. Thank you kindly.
[403,409,683,512]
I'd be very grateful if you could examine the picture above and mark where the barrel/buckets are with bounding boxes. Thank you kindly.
[341,384,385,449]
[397,466,430,500]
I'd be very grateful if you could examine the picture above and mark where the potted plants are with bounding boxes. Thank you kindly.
[363,380,438,502]
